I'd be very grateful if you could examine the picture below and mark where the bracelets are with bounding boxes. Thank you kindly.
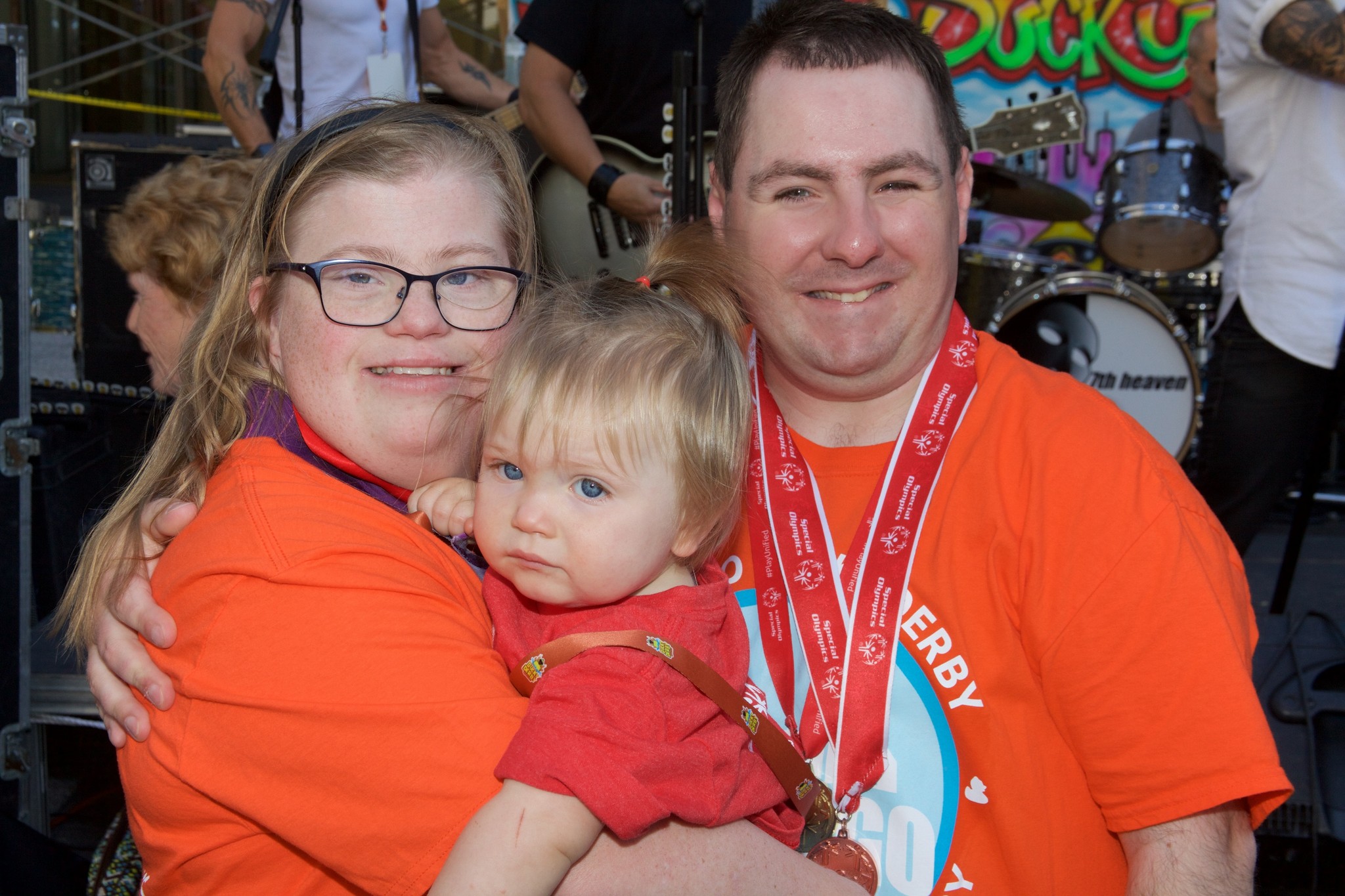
[587,163,626,206]
[251,141,277,159]
[508,88,518,103]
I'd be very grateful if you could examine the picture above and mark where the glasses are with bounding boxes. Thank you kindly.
[266,257,531,333]
[1194,54,1216,72]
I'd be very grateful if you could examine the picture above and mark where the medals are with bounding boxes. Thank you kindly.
[804,806,878,896]
[794,756,836,852]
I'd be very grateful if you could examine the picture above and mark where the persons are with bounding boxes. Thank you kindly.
[202,0,515,158]
[107,159,401,511]
[87,0,1294,896]
[512,0,755,304]
[405,227,832,896]
[1187,0,1345,560]
[94,105,875,896]
[1119,17,1230,213]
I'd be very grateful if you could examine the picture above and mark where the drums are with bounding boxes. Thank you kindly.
[954,243,1083,330]
[1095,137,1229,271]
[983,270,1201,466]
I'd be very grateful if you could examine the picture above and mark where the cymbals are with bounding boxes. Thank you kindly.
[967,160,1091,221]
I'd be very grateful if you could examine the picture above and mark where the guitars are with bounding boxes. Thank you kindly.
[479,74,586,134]
[527,87,1086,289]
